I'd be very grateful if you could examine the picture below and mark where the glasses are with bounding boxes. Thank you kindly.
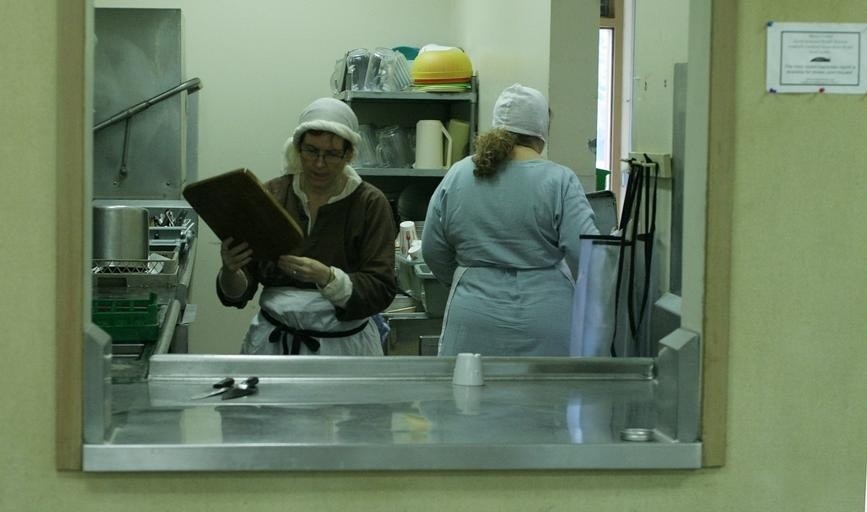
[298,143,348,165]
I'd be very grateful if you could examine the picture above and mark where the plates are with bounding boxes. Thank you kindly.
[411,84,469,93]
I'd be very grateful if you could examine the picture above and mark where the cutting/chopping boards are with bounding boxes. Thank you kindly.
[183,168,304,258]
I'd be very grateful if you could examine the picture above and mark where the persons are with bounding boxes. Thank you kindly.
[218,97,397,358]
[419,85,610,359]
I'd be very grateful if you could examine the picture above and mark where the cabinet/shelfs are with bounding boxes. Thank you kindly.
[338,88,473,178]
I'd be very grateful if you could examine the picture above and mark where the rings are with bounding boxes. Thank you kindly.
[291,270,297,276]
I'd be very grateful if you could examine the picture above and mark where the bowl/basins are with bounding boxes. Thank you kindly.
[412,48,473,84]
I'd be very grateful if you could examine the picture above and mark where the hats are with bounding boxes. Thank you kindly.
[281,97,363,174]
[491,81,550,143]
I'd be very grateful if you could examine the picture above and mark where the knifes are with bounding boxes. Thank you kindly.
[189,375,259,400]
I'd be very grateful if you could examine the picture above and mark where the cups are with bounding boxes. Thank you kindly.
[346,48,395,93]
[452,353,485,386]
[374,118,470,169]
[407,245,423,261]
[400,220,419,255]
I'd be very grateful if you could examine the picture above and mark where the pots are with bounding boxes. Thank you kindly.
[92,204,149,270]
